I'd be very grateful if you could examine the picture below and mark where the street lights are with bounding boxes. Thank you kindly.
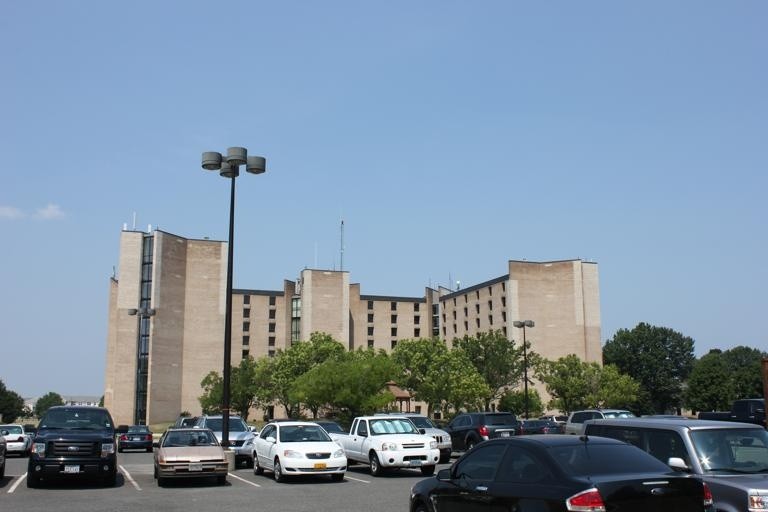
[126,307,157,425]
[200,147,266,473]
[511,319,535,418]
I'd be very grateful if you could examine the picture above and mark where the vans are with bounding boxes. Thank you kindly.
[566,410,638,438]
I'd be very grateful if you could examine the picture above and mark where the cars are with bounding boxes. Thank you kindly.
[252,420,349,482]
[0,420,32,484]
[519,415,566,437]
[175,416,257,466]
[155,427,228,490]
[408,434,716,512]
[119,426,154,453]
[580,416,767,511]
[315,420,343,434]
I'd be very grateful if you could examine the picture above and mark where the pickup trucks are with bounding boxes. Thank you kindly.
[327,416,439,476]
[697,397,767,432]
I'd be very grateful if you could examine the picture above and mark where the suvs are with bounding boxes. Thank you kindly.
[447,411,520,455]
[27,405,116,490]
[375,412,452,467]
